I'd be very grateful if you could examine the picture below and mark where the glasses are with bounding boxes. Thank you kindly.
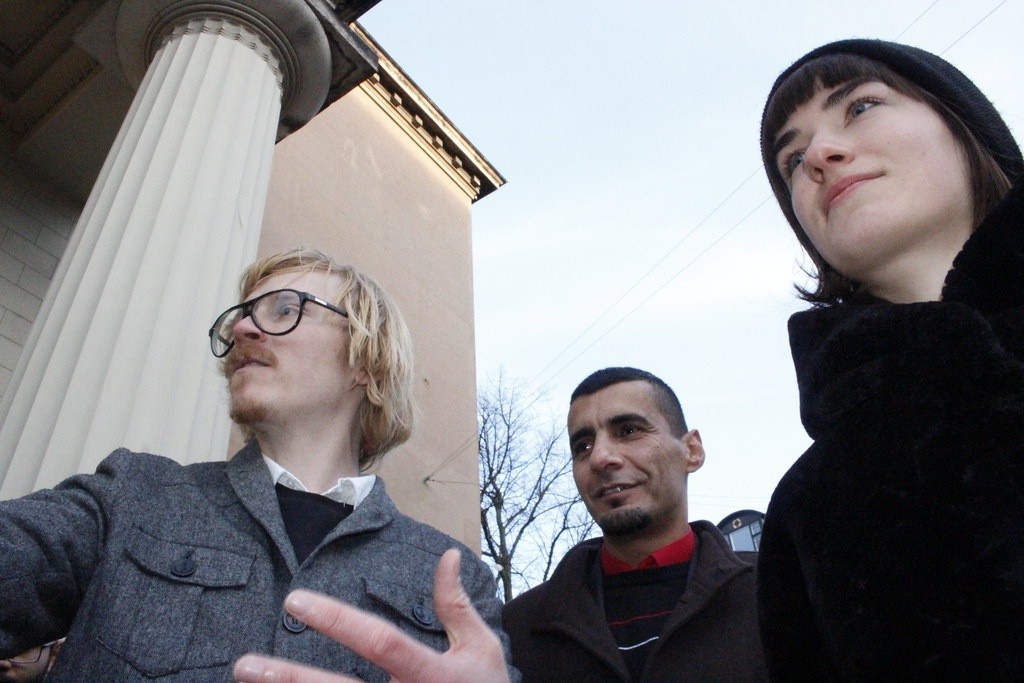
[8,641,57,663]
[208,289,348,358]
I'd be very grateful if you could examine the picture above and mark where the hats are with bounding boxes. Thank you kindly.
[761,39,1024,273]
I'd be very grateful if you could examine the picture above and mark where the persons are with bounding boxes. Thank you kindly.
[502,367,769,683]
[0,243,522,683]
[757,40,1024,683]
